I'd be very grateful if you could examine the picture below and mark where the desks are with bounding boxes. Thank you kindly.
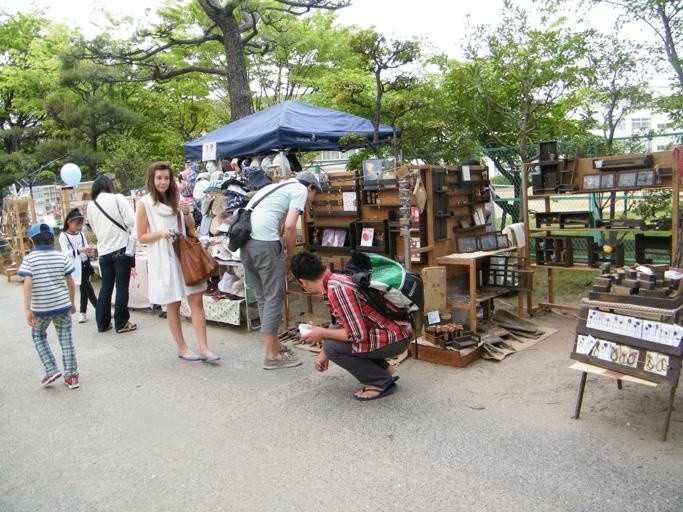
[574,297,683,442]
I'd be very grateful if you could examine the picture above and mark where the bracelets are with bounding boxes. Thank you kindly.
[184,212,189,215]
[321,328,323,338]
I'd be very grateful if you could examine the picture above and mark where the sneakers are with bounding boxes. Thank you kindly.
[77,313,86,323]
[62,374,80,389]
[115,319,137,334]
[98,320,112,333]
[39,371,62,386]
[262,351,303,371]
[279,343,295,355]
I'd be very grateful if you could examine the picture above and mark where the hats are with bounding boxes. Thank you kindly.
[208,215,225,236]
[217,271,238,294]
[223,194,248,213]
[230,276,248,299]
[200,199,215,216]
[210,197,229,216]
[198,214,211,236]
[294,171,323,194]
[27,221,54,241]
[178,151,290,198]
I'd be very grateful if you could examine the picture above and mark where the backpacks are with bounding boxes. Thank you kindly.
[330,249,425,323]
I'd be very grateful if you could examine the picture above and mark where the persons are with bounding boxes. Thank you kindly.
[135,161,221,362]
[59,207,97,324]
[291,251,410,401]
[87,175,137,334]
[239,171,323,370]
[18,222,79,389]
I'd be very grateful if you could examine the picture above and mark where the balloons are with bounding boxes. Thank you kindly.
[60,162,82,186]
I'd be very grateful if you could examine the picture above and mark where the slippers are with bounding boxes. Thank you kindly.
[352,383,397,401]
[391,374,401,382]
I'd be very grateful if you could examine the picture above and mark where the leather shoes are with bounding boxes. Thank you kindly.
[175,351,206,361]
[205,353,222,364]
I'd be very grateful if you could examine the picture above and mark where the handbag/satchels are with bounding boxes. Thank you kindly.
[221,208,253,253]
[170,229,220,288]
